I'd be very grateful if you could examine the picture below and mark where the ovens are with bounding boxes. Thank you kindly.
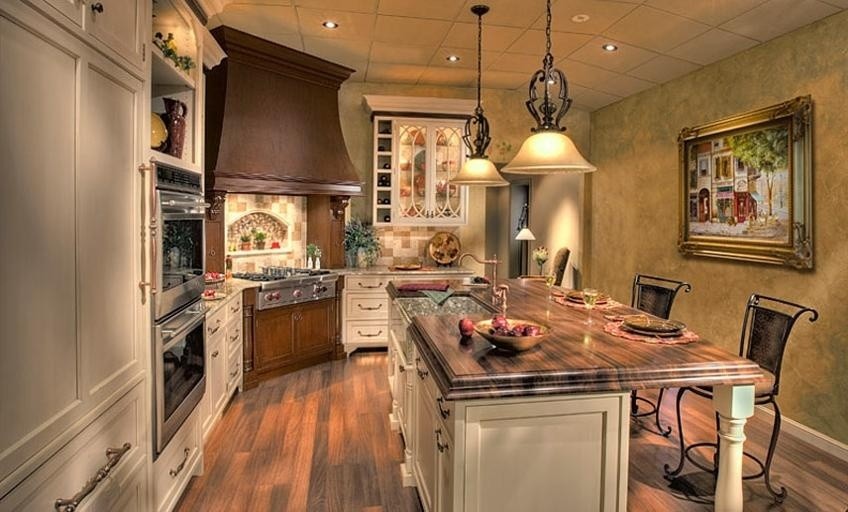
[150,295,207,463]
[150,157,206,321]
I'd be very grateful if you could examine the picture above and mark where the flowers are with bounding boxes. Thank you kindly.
[305,242,315,257]
[339,217,384,273]
[313,246,323,258]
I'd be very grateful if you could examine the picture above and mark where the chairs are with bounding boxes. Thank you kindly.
[664,293,818,503]
[632,271,691,436]
[547,247,571,285]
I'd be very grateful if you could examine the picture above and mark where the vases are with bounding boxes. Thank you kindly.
[355,247,367,271]
[315,256,321,269]
[307,254,313,269]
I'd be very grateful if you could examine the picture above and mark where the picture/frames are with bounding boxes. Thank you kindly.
[677,94,815,273]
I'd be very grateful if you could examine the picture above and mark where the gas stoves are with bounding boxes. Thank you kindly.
[232,265,340,312]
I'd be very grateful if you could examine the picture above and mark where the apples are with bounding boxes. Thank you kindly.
[459,318,474,337]
[508,330,522,337]
[525,326,540,336]
[512,323,530,334]
[492,315,509,330]
[493,327,509,336]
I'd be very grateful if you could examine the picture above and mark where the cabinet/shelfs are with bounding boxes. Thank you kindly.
[239,275,342,392]
[202,286,243,440]
[1,0,144,512]
[340,266,472,362]
[385,277,775,512]
[148,409,205,512]
[151,0,206,167]
[364,95,471,226]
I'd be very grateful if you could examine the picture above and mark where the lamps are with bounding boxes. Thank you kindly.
[516,204,537,244]
[453,4,508,188]
[499,0,598,176]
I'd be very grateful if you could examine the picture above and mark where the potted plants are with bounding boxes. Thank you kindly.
[239,232,251,250]
[254,232,267,249]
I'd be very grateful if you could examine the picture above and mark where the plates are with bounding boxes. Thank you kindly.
[428,230,462,265]
[620,324,685,337]
[623,314,686,333]
[472,317,550,353]
[394,263,422,272]
[563,294,612,304]
[149,110,169,148]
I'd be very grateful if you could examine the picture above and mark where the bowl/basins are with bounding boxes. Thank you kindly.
[441,163,459,172]
[439,188,456,197]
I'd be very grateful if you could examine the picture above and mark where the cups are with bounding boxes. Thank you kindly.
[543,273,557,303]
[581,289,597,325]
